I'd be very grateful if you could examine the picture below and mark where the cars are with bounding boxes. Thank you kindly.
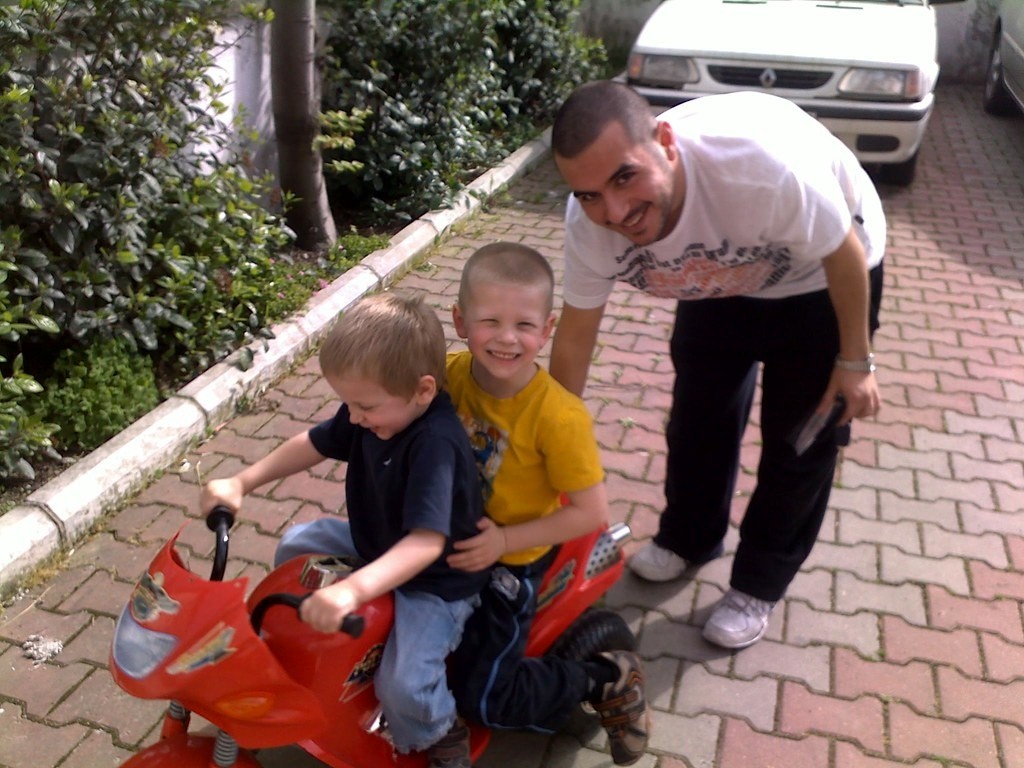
[980,0,1024,116]
[626,0,940,190]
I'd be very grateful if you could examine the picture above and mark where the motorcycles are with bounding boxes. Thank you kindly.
[110,501,630,767]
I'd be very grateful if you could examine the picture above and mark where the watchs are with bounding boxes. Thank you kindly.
[836,352,877,374]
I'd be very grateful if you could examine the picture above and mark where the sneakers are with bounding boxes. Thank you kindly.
[431,719,470,768]
[588,651,651,765]
[700,588,779,650]
[629,540,687,582]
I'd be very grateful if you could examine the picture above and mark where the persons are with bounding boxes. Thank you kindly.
[438,240,654,768]
[547,76,891,654]
[197,290,490,768]
